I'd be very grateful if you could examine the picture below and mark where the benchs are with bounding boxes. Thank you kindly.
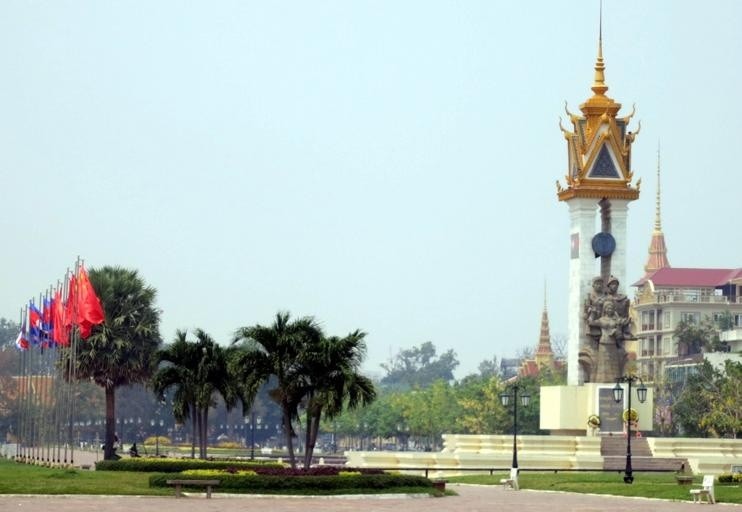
[689,474,717,505]
[500,468,520,490]
[167,479,222,499]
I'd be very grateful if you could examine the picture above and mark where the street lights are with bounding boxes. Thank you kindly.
[612,373,647,484]
[499,382,531,488]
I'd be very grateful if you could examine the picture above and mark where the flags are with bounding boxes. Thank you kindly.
[14,267,104,352]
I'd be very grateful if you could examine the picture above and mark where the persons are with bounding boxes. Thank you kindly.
[131,442,138,456]
[582,277,606,352]
[603,276,630,350]
[589,300,633,381]
